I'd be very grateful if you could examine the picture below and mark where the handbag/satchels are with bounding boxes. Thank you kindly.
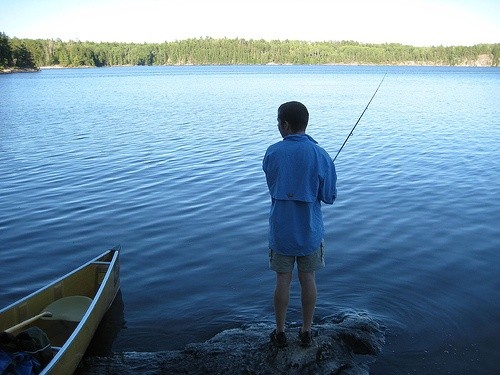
[0,325,54,375]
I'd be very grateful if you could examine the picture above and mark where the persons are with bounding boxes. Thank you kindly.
[261,101,337,349]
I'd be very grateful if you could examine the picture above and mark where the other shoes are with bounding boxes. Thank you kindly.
[270,328,289,349]
[299,327,313,346]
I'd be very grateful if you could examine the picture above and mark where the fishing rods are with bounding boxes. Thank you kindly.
[332,71,388,161]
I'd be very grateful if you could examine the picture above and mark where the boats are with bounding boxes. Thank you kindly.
[0,244,123,375]
